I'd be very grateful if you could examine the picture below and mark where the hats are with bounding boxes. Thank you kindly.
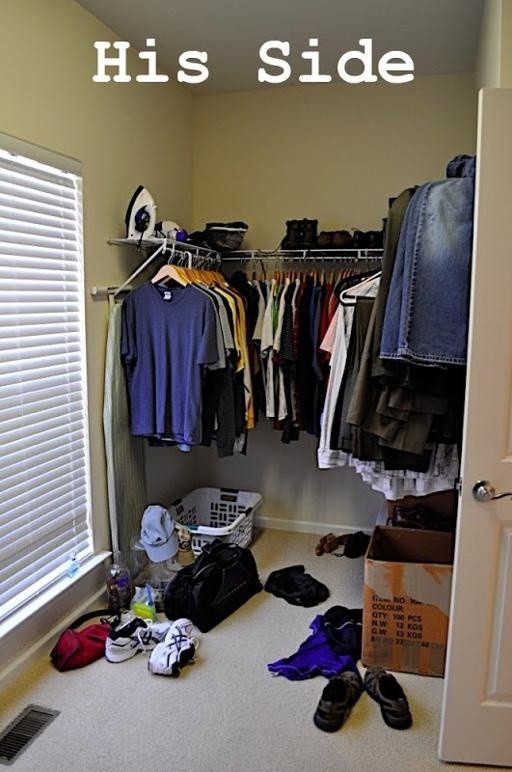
[141,505,195,566]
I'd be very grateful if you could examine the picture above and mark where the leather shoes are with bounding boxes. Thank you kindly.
[281,219,383,256]
[392,503,449,531]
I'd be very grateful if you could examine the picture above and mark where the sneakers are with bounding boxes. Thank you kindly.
[148,618,197,679]
[106,614,171,663]
[365,668,413,731]
[313,671,363,733]
[343,530,371,559]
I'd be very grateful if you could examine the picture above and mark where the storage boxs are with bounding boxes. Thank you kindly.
[359,525,454,678]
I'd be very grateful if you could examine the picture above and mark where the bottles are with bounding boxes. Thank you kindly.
[105,563,135,613]
[68,551,81,579]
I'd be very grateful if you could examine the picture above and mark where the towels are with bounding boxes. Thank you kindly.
[322,603,364,656]
[267,612,360,681]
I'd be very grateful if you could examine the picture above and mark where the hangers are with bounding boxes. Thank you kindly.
[160,243,385,296]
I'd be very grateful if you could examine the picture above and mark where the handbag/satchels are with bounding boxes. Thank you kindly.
[52,621,114,671]
[163,538,263,633]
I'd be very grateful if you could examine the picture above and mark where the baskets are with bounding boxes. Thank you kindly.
[166,487,268,557]
